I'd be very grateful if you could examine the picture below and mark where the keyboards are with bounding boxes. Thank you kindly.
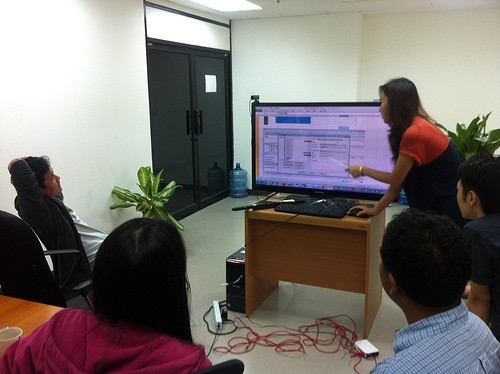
[274,202,349,218]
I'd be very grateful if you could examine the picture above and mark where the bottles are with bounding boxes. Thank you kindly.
[229,163,248,198]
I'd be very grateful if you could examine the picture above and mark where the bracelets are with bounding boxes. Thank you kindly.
[360,165,366,175]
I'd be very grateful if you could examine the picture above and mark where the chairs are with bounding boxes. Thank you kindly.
[0,210,246,374]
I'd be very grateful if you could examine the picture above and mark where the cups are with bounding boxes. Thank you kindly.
[0,327,22,357]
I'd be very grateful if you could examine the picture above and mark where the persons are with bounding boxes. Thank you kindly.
[0,217,214,374]
[7,156,110,290]
[345,77,500,374]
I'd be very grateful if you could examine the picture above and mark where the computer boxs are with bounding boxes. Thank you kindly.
[226,246,247,313]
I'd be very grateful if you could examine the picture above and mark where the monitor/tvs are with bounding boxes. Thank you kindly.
[252,101,399,207]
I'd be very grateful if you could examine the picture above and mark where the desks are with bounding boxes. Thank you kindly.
[246,192,386,342]
[0,294,65,357]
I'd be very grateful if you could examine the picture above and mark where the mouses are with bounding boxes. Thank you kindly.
[349,208,368,218]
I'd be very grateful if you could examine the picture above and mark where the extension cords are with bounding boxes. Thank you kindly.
[213,300,222,329]
